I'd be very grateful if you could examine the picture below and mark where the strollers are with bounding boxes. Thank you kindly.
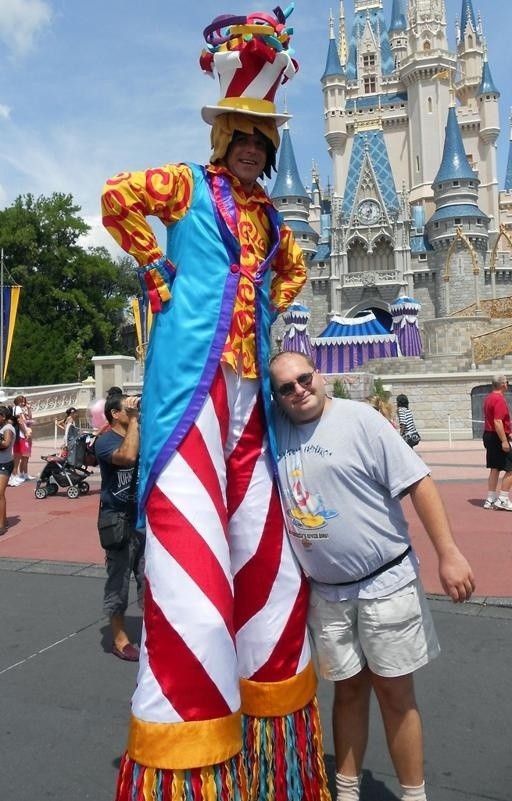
[35,423,95,499]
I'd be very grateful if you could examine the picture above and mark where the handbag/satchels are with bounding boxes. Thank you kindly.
[403,431,421,447]
[97,511,131,549]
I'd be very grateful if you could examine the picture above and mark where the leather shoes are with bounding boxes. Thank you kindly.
[112,642,140,662]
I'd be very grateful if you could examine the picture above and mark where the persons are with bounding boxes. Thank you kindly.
[20,405,37,481]
[55,406,82,445]
[0,405,17,535]
[260,349,481,801]
[482,373,511,514]
[6,396,26,488]
[395,393,419,450]
[100,129,312,777]
[93,393,145,664]
[92,386,121,440]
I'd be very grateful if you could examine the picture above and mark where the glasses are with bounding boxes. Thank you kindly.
[272,369,315,397]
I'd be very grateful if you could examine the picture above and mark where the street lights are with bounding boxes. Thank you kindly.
[76,352,84,383]
[275,334,283,353]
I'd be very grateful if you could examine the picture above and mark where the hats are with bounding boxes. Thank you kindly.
[200,4,300,164]
[106,387,122,395]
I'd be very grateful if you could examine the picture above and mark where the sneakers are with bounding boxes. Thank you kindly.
[7,474,36,488]
[494,497,512,512]
[483,499,497,510]
